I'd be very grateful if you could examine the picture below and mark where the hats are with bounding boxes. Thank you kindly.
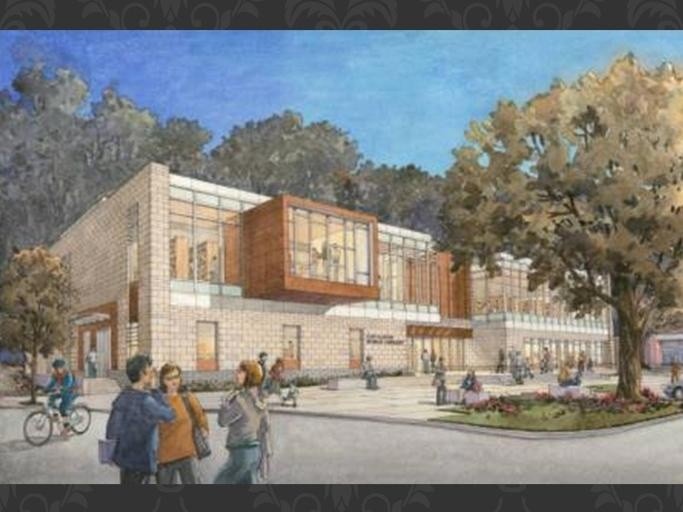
[51,358,66,367]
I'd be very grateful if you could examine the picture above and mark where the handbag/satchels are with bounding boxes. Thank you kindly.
[181,392,212,460]
[98,437,117,467]
[432,378,443,388]
[258,429,272,480]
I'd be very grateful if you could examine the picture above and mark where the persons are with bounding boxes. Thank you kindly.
[419,344,592,405]
[211,359,271,484]
[150,362,210,484]
[84,343,100,378]
[40,358,81,436]
[669,365,681,384]
[256,351,270,404]
[267,358,283,397]
[103,353,176,484]
[278,374,300,407]
[329,242,342,282]
[309,246,321,277]
[361,353,380,389]
